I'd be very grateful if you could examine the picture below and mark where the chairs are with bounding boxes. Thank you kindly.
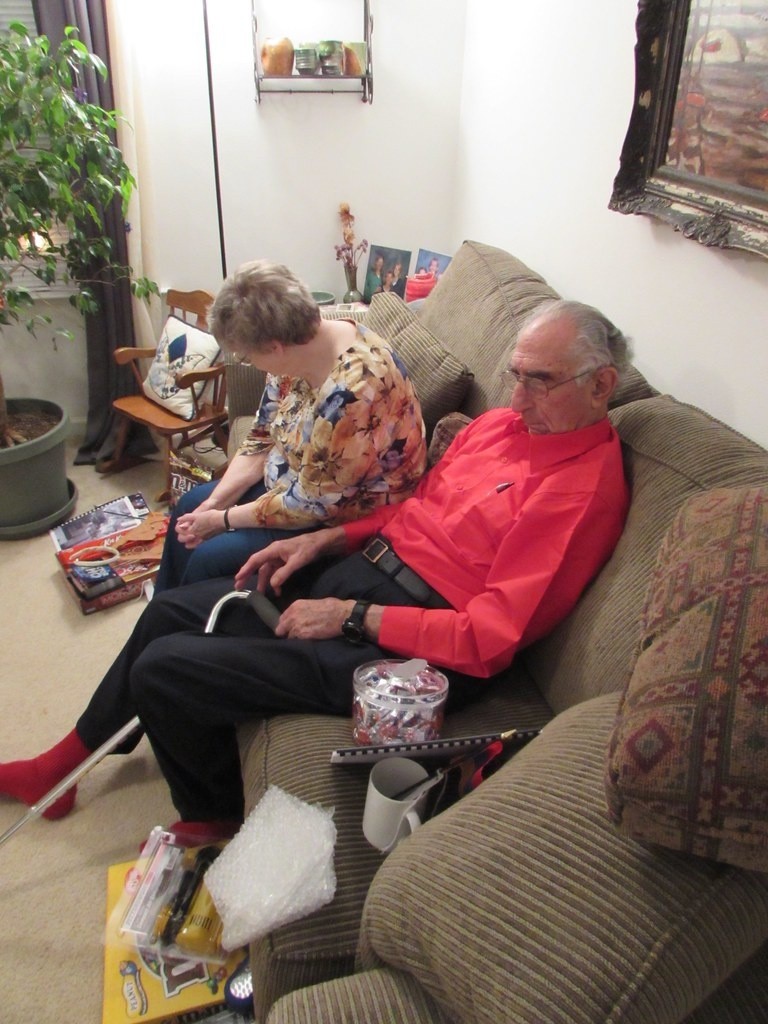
[94,287,231,505]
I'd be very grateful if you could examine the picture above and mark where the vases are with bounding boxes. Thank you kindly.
[343,265,363,304]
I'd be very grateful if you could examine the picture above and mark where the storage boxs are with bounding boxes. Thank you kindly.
[52,521,160,615]
[102,838,254,1024]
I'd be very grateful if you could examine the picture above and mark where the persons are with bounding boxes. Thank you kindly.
[391,256,405,298]
[1,299,635,855]
[150,259,428,588]
[374,268,395,294]
[417,268,428,273]
[364,253,384,302]
[428,257,444,281]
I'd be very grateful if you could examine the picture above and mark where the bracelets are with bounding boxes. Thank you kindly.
[224,504,239,533]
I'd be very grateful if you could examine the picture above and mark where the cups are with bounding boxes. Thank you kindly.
[294,48,317,76]
[362,757,430,854]
[261,38,294,78]
[343,42,366,76]
[318,41,344,76]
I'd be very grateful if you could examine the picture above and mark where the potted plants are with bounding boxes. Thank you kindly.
[1,22,163,540]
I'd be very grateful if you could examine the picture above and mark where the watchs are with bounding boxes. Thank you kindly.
[341,600,374,645]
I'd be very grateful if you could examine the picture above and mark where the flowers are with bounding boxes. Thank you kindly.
[334,200,368,270]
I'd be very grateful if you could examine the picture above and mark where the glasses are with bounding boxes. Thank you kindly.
[232,344,258,368]
[503,370,594,400]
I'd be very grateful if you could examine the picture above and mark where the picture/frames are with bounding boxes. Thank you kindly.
[607,0,768,260]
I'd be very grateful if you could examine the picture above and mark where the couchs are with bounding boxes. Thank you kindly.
[220,239,767,1024]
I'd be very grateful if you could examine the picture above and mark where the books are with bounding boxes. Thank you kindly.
[50,491,175,615]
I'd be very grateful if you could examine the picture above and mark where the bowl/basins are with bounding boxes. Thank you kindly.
[311,291,335,305]
[352,660,449,751]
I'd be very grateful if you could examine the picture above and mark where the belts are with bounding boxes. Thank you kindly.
[362,537,450,609]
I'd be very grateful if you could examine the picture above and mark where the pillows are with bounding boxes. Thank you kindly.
[142,315,221,421]
[359,291,473,450]
[603,481,767,872]
